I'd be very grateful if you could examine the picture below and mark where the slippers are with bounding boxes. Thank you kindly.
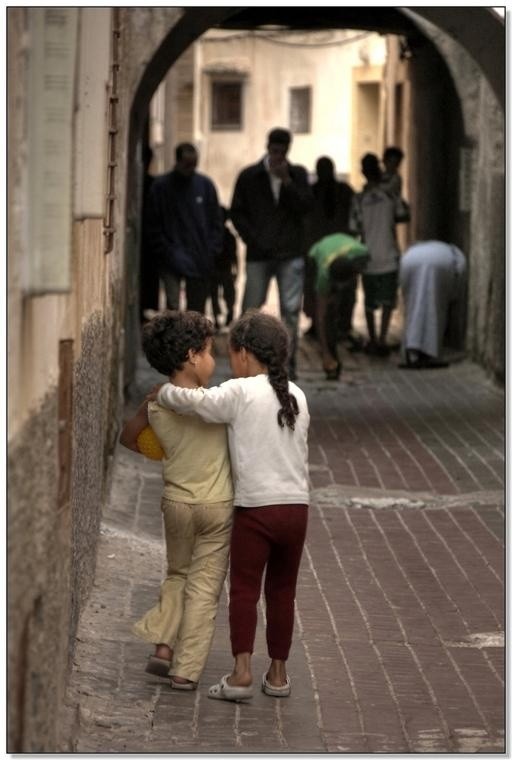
[263,670,292,696]
[209,673,253,700]
[146,655,198,690]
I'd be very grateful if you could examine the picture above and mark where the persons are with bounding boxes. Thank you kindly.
[204,203,238,329]
[307,233,372,380]
[229,127,314,382]
[117,307,238,694]
[354,146,405,233]
[149,312,311,704]
[398,240,466,370]
[146,143,219,313]
[140,144,159,322]
[349,153,411,357]
[303,155,359,340]
